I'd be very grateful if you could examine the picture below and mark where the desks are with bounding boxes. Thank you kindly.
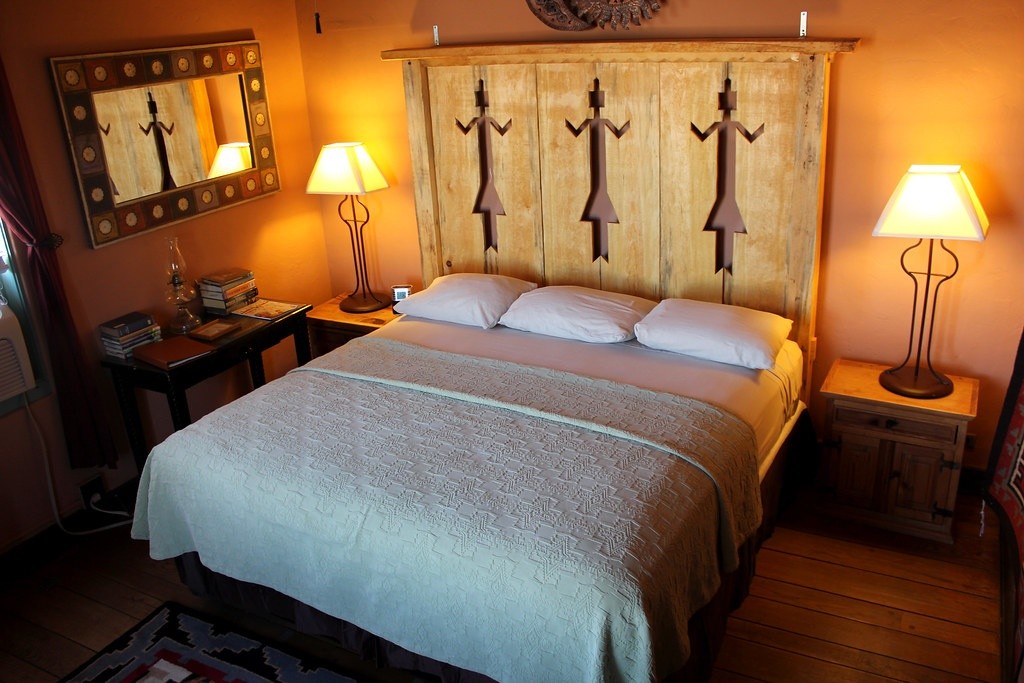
[100,297,315,482]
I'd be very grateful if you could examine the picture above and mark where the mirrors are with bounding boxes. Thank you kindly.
[47,34,282,250]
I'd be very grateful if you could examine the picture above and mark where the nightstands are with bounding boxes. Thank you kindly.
[818,353,981,545]
[303,292,400,357]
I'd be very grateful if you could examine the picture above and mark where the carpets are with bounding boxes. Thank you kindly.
[50,598,372,682]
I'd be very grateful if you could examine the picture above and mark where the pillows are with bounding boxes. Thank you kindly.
[634,297,795,373]
[392,273,539,329]
[499,285,659,345]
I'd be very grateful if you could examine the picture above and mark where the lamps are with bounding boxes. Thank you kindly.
[868,163,991,402]
[304,142,394,314]
[205,141,252,178]
[164,235,203,336]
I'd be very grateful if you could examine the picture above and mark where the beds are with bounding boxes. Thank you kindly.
[131,313,807,683]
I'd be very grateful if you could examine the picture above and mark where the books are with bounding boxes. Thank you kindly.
[131,336,216,370]
[232,298,299,321]
[200,267,259,318]
[98,311,163,360]
